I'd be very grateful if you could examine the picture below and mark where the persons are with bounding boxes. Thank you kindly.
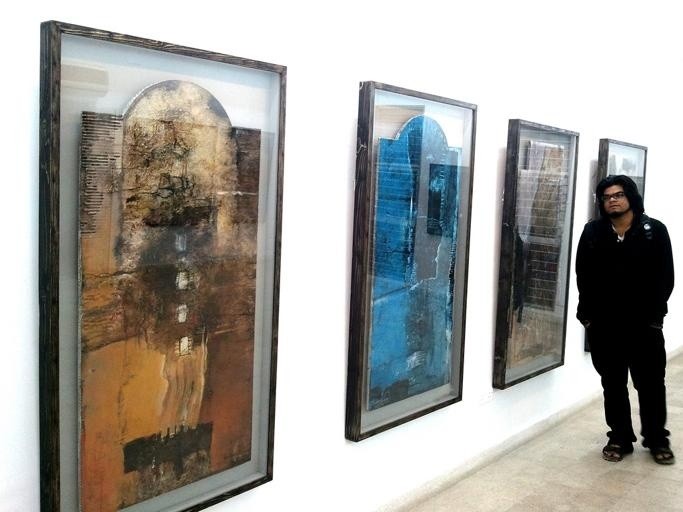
[574,173,677,466]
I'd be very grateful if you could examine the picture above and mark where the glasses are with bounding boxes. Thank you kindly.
[603,192,624,203]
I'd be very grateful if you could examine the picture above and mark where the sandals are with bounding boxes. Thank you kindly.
[602,440,634,462]
[649,446,674,465]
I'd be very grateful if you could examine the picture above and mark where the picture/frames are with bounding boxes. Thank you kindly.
[345,81,478,442]
[492,118,580,390]
[596,138,647,223]
[38,19,288,512]
